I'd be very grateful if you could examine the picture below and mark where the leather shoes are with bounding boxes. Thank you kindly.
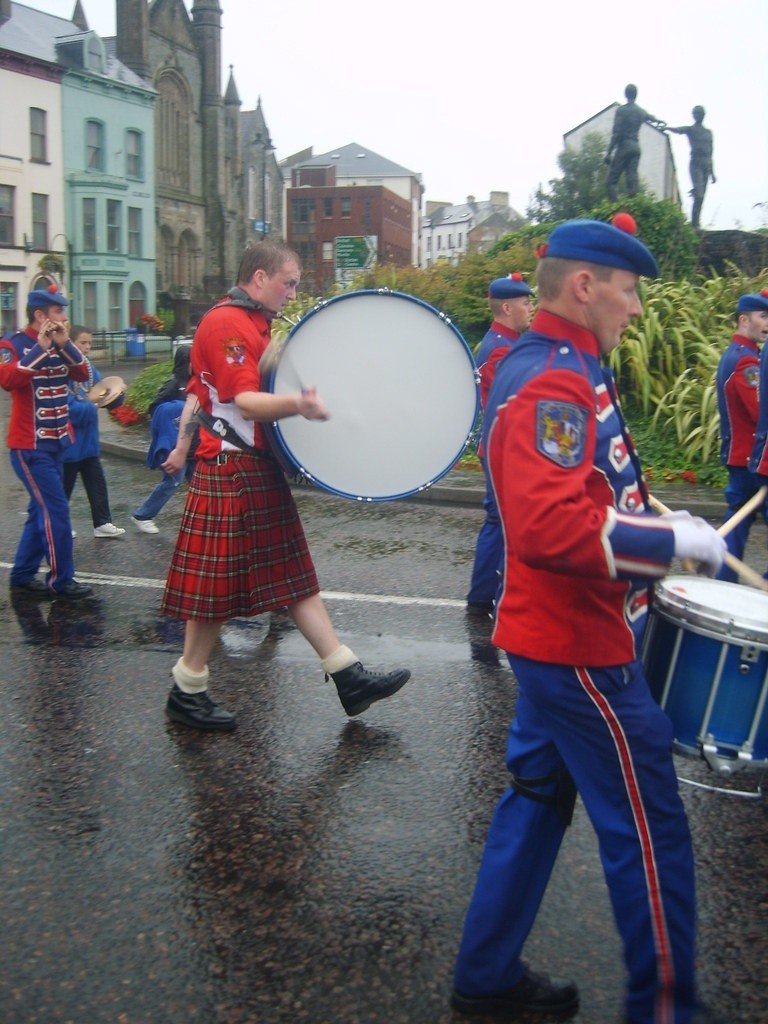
[450,962,578,1015]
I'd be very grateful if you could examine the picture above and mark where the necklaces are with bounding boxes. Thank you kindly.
[79,358,92,392]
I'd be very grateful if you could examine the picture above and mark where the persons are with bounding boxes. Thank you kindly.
[0,285,93,597]
[161,241,412,733]
[466,272,536,616]
[716,288,768,582]
[447,212,719,1024]
[604,84,666,200]
[161,377,200,476]
[129,345,193,533]
[63,325,125,537]
[656,106,716,230]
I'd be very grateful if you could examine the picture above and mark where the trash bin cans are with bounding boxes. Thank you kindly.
[124,326,147,356]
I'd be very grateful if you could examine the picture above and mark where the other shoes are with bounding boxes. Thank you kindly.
[466,601,495,615]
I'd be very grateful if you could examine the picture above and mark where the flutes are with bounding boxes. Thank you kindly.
[45,325,58,333]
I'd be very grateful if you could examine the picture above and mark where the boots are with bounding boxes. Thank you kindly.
[165,683,235,729]
[329,661,411,716]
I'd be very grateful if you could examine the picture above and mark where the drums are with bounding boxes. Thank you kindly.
[635,574,768,771]
[260,285,483,505]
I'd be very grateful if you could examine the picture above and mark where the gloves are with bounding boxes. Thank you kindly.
[669,514,728,576]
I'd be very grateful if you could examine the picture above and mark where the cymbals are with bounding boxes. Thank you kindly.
[90,375,124,408]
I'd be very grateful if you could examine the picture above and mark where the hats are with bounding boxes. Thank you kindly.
[736,290,768,311]
[487,273,535,299]
[536,213,659,279]
[26,286,68,306]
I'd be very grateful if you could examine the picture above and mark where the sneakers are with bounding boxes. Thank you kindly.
[10,579,50,595]
[72,530,76,538]
[94,522,126,537]
[131,516,159,533]
[52,580,91,599]
